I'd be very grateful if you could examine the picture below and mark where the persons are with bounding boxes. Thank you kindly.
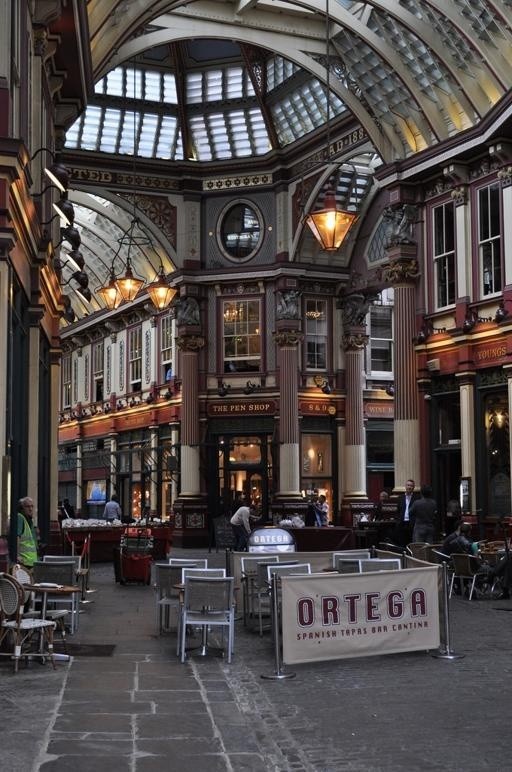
[230,503,255,553]
[375,491,389,521]
[451,524,472,554]
[61,499,75,519]
[397,480,417,546]
[409,485,438,544]
[17,496,38,574]
[444,520,464,550]
[103,494,122,522]
[317,494,329,527]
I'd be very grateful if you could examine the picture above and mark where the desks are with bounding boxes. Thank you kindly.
[173,582,186,590]
[22,582,81,664]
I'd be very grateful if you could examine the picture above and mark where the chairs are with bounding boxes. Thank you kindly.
[42,556,88,599]
[431,547,450,562]
[154,563,198,634]
[326,546,375,568]
[406,541,427,558]
[337,557,361,574]
[28,561,77,636]
[359,557,401,572]
[240,555,280,627]
[12,563,69,659]
[181,567,226,591]
[178,576,235,664]
[267,563,312,608]
[446,553,487,601]
[251,559,298,629]
[418,545,443,558]
[0,572,57,672]
[169,557,208,571]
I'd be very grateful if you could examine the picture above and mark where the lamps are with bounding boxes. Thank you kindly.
[29,184,75,227]
[52,227,81,250]
[28,148,69,192]
[60,270,89,288]
[298,163,359,253]
[54,251,86,272]
[94,221,177,311]
[64,284,92,305]
